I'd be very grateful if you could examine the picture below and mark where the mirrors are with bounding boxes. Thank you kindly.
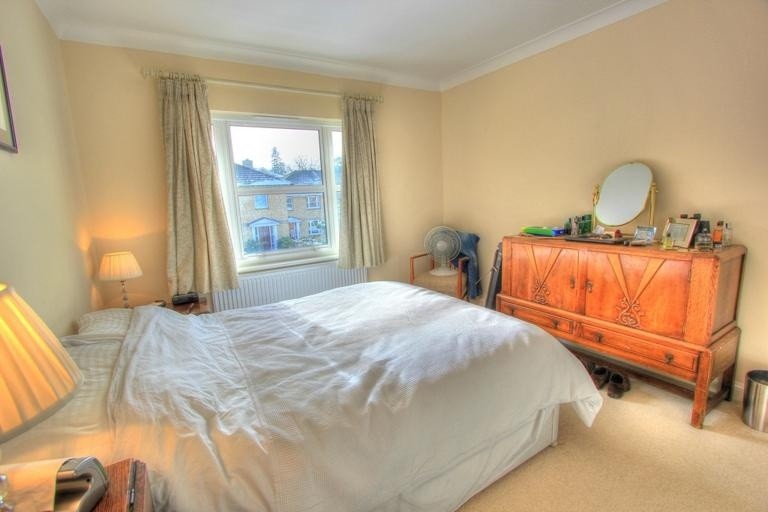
[591,160,658,244]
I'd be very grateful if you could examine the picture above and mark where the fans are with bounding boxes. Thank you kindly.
[423,226,462,276]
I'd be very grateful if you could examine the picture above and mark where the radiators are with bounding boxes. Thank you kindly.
[207,261,367,313]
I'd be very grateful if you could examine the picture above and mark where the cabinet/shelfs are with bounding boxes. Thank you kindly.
[493,233,748,430]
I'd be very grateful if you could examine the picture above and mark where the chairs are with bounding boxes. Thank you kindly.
[409,229,480,302]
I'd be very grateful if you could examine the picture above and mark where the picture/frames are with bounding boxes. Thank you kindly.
[661,217,698,248]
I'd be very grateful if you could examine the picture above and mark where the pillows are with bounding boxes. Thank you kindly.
[39,308,136,439]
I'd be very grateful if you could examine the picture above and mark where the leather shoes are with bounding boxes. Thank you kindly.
[607,371,631,400]
[591,367,611,391]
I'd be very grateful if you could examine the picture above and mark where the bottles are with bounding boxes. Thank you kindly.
[722,223,731,247]
[663,233,674,249]
[694,228,713,251]
[712,221,723,248]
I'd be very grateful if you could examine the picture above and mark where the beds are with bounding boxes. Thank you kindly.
[7,276,569,512]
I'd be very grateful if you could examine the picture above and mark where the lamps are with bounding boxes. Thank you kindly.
[97,250,145,307]
[2,282,85,507]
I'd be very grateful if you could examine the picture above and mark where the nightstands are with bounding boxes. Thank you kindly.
[159,292,211,316]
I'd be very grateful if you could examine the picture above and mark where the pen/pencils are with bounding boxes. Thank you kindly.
[130,462,136,511]
[185,302,195,314]
